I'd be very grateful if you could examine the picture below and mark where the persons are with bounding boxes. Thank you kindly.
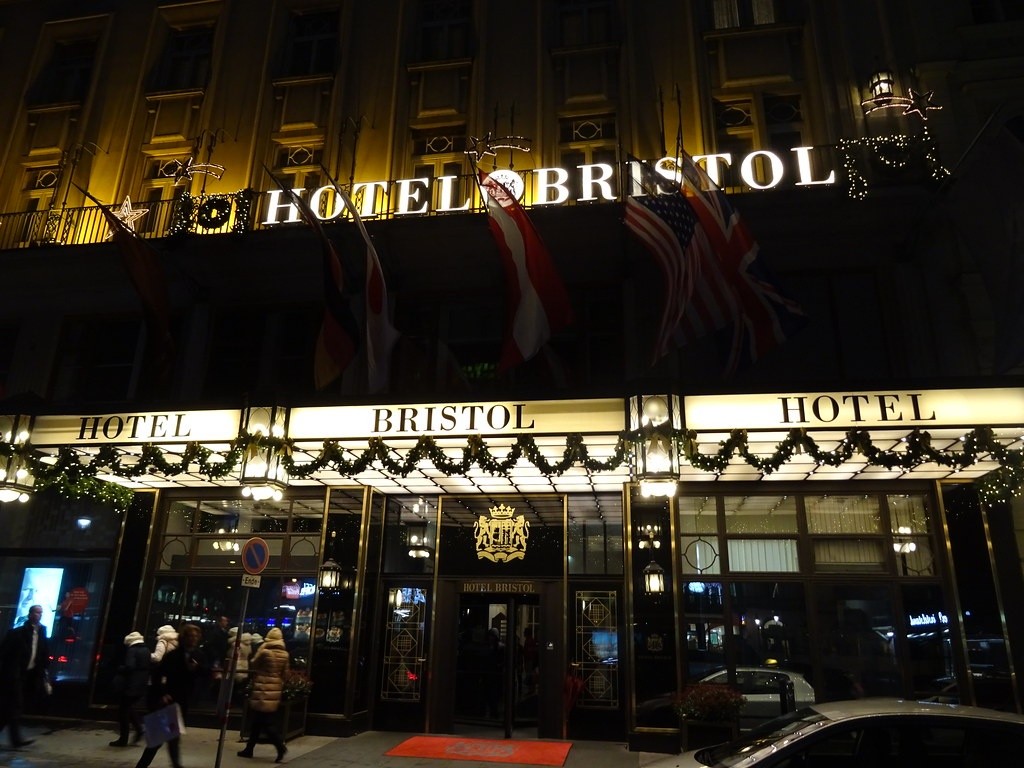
[844,664,891,756]
[207,616,264,708]
[0,606,49,748]
[479,628,506,719]
[513,627,538,696]
[110,624,204,768]
[236,627,289,763]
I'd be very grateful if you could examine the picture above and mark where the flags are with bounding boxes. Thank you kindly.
[619,148,802,381]
[479,169,575,382]
[103,212,171,403]
[293,184,398,396]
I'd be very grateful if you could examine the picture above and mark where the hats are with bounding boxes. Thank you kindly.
[228,627,238,636]
[265,627,282,641]
[124,631,144,645]
[157,624,175,635]
[252,634,263,643]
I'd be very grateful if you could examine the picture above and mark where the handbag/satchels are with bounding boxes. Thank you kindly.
[142,698,186,748]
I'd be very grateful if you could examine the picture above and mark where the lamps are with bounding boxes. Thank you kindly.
[638,549,667,598]
[238,442,294,501]
[403,520,435,559]
[864,55,895,108]
[74,511,95,531]
[315,551,346,600]
[633,430,685,498]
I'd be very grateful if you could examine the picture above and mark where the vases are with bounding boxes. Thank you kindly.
[240,692,312,744]
[678,717,739,753]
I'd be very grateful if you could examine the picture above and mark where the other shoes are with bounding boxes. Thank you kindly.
[238,749,253,757]
[15,740,33,748]
[275,745,287,762]
[110,740,127,748]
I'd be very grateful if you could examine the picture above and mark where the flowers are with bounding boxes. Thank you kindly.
[670,679,748,725]
[247,667,316,699]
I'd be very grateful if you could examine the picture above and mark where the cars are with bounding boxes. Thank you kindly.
[634,668,815,736]
[641,699,1024,768]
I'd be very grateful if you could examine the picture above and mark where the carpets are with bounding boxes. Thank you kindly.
[384,733,574,768]
